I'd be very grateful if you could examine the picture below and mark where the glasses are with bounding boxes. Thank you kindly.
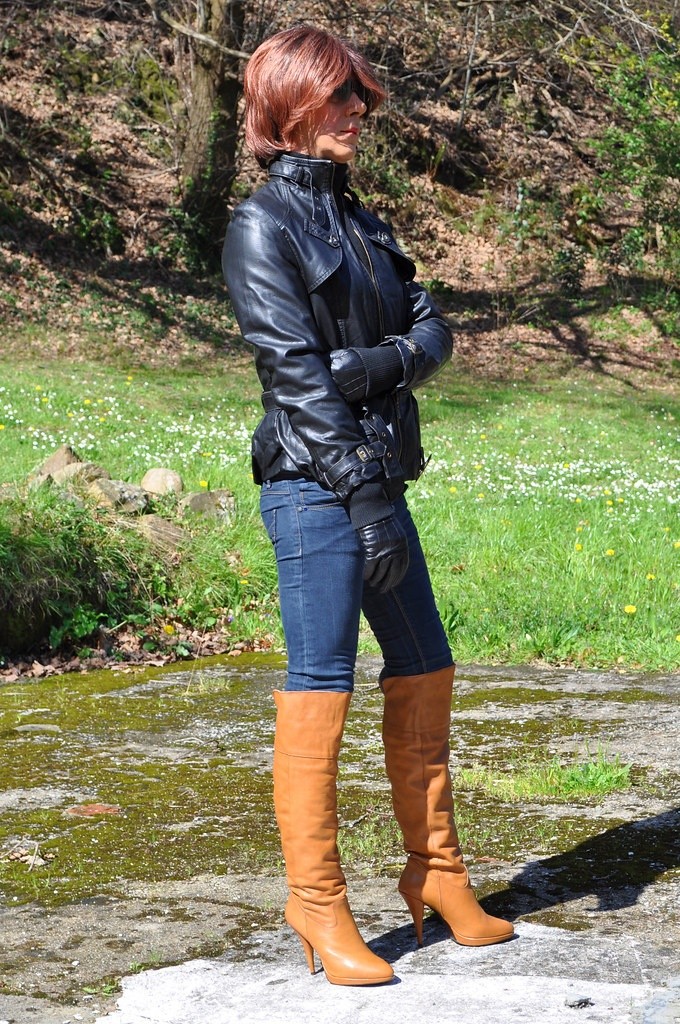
[327,80,373,121]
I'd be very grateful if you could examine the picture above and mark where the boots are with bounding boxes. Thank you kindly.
[272,689,395,986]
[382,664,514,947]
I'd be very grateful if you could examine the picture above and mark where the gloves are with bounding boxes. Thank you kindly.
[356,515,410,593]
[323,349,368,403]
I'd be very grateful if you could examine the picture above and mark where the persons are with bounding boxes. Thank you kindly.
[219,25,514,986]
